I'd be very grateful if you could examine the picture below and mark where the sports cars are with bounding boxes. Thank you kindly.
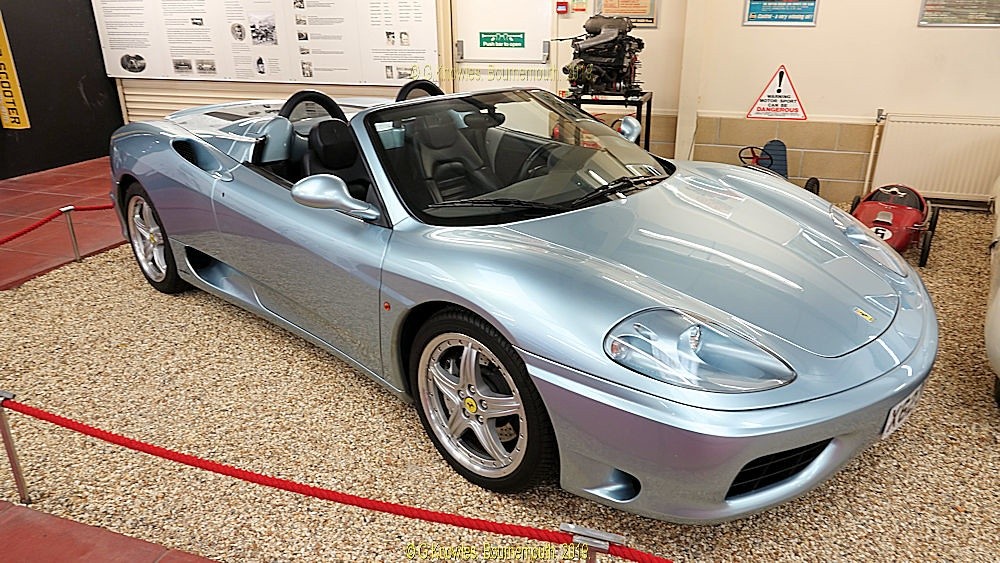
[111,86,939,526]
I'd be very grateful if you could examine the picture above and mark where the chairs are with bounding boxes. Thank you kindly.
[412,112,497,205]
[304,117,370,201]
